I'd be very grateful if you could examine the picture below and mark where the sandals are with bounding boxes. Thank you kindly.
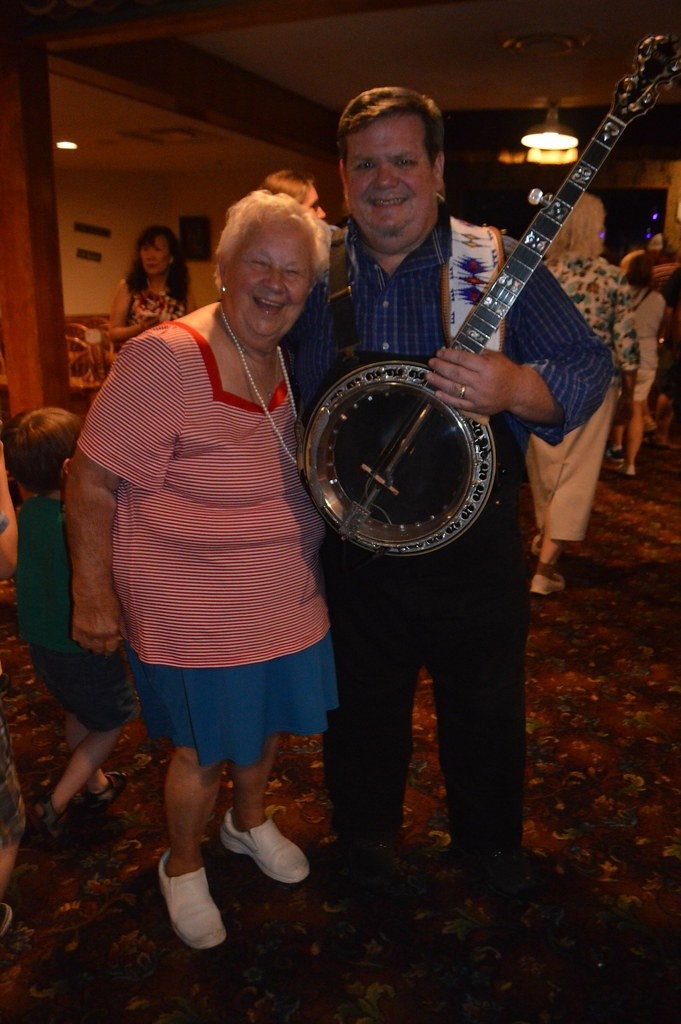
[29,793,71,850]
[83,768,128,813]
[652,440,681,450]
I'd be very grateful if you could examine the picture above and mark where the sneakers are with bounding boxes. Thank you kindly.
[604,443,625,464]
[323,832,389,905]
[158,845,228,951]
[447,840,546,899]
[220,806,310,885]
[621,462,637,477]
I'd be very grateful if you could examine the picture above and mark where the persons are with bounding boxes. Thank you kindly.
[109,223,189,344]
[0,405,141,943]
[65,171,340,952]
[277,88,640,896]
[259,169,325,219]
[609,234,681,478]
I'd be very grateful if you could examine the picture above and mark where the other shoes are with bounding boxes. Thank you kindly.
[527,572,566,596]
[532,537,542,559]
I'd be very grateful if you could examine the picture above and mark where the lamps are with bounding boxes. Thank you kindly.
[521,108,578,150]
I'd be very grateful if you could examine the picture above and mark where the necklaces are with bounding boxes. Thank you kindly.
[220,306,297,465]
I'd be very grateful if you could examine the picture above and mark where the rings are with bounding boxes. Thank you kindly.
[460,384,465,399]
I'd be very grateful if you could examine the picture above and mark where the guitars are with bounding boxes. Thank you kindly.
[290,32,679,557]
